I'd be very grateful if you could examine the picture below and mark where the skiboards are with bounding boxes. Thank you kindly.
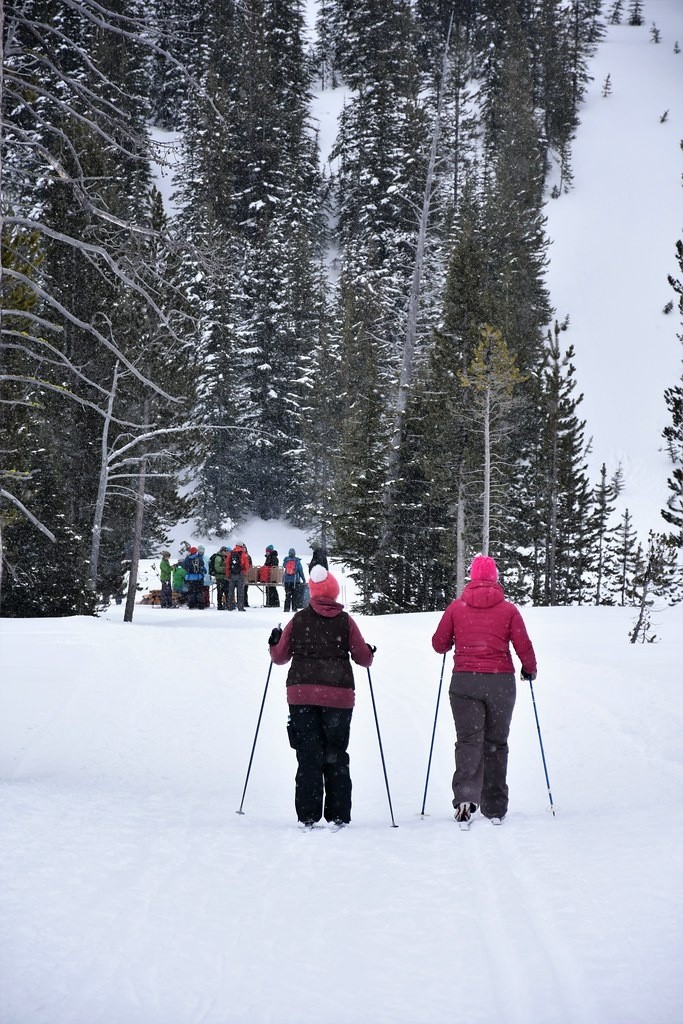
[299,820,346,833]
[456,817,507,832]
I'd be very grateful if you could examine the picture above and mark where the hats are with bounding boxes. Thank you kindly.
[189,546,198,554]
[469,556,498,583]
[196,544,205,552]
[288,548,295,554]
[219,546,228,551]
[266,545,274,552]
[308,564,340,598]
[309,542,320,548]
[235,540,244,547]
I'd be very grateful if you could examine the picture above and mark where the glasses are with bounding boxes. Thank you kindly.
[224,550,228,552]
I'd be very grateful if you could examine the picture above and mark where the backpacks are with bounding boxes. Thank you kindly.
[209,553,224,576]
[230,550,244,574]
[171,565,190,593]
[285,557,297,575]
[187,555,201,574]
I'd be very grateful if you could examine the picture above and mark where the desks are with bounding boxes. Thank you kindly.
[205,584,288,607]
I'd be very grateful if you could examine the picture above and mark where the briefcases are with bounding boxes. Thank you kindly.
[292,582,310,607]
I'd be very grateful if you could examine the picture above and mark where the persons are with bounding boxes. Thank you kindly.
[268,566,377,830]
[159,541,328,612]
[432,556,537,821]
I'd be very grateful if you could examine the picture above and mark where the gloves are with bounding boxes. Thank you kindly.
[520,667,537,681]
[268,628,283,645]
[366,642,377,652]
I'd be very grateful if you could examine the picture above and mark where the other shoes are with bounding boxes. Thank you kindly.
[218,603,236,611]
[284,608,290,612]
[453,801,479,822]
[238,608,246,611]
[292,607,298,612]
